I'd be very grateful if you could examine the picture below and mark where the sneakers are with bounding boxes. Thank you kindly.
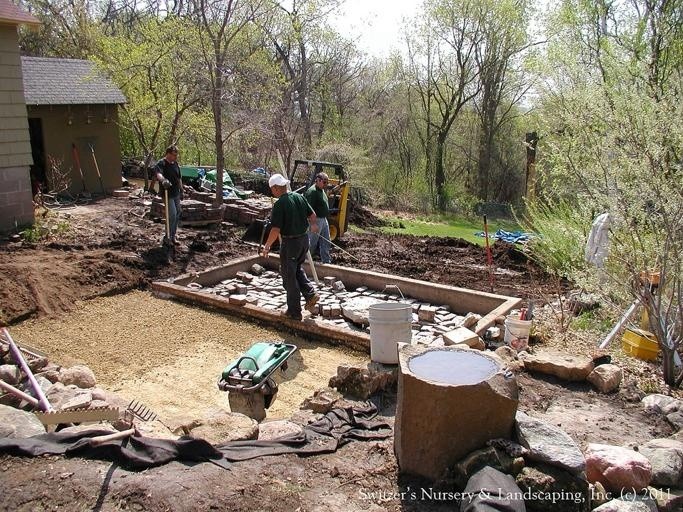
[305,293,319,310]
[165,236,181,246]
[285,311,302,320]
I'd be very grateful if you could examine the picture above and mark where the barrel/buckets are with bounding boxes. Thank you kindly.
[504,315,532,350]
[369,285,412,365]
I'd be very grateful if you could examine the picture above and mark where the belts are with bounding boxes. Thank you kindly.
[282,232,307,238]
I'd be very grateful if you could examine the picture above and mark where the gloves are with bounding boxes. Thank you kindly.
[162,179,172,188]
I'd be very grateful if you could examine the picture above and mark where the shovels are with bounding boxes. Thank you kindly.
[89,421,142,448]
[484,214,499,295]
[163,184,175,261]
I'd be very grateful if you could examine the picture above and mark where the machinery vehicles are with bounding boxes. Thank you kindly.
[240,159,351,251]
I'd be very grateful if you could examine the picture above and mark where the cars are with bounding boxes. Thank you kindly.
[250,167,270,178]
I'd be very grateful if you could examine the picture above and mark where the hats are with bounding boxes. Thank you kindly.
[268,173,290,188]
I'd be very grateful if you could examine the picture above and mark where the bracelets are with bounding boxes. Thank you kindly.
[264,247,271,251]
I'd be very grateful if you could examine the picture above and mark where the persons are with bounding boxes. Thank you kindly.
[305,172,332,264]
[154,146,184,246]
[262,173,320,322]
